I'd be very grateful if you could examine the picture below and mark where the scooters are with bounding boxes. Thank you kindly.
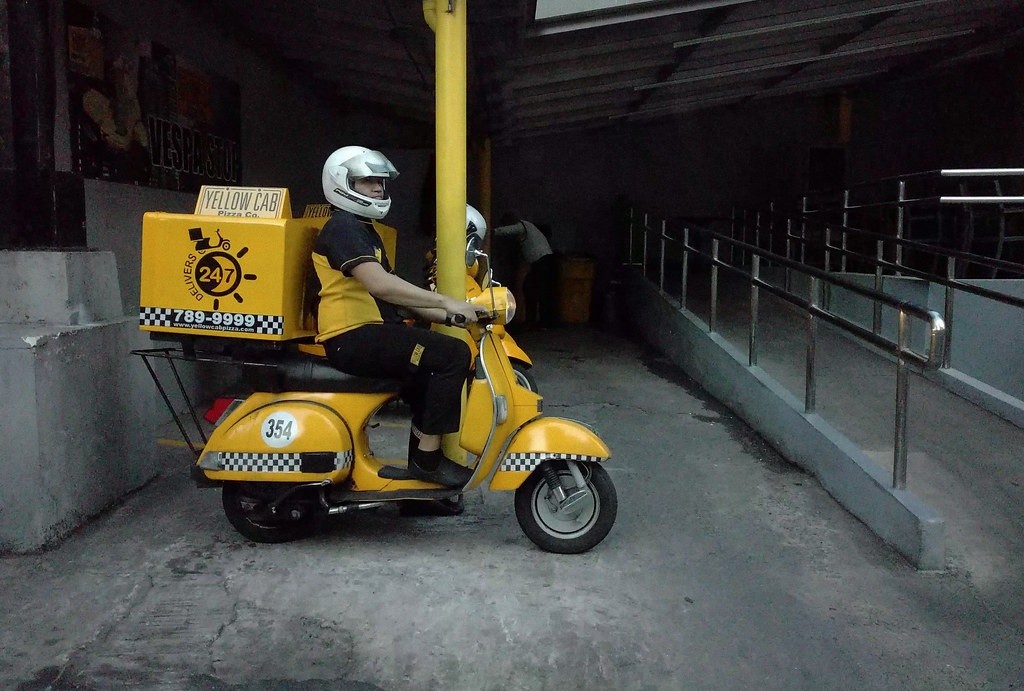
[130,236,618,554]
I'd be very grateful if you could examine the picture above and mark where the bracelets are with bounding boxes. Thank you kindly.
[445,312,454,327]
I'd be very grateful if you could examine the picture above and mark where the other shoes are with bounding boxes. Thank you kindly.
[408,453,474,488]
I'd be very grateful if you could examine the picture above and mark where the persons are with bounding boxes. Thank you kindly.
[311,146,479,515]
[487,208,562,330]
[423,203,488,293]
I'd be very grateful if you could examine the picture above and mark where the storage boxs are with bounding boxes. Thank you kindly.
[138,213,399,341]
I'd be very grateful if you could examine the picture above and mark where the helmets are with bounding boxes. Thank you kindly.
[321,146,401,220]
[466,203,488,241]
